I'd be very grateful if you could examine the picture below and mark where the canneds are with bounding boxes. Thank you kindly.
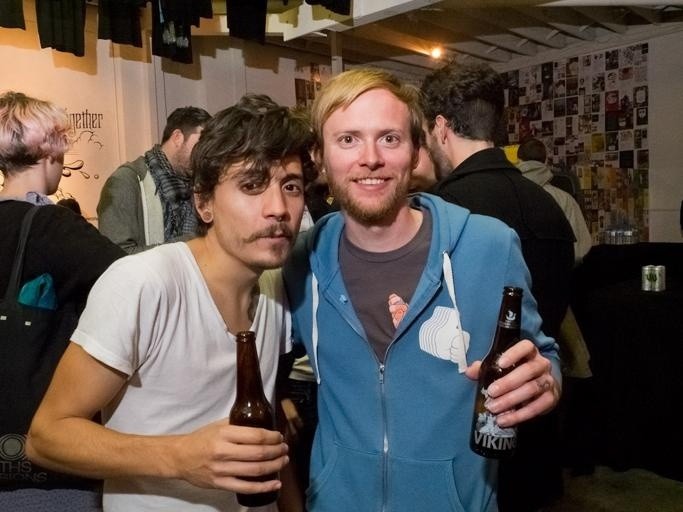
[641,264,667,292]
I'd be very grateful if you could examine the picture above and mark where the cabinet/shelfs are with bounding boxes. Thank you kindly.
[556,239,681,485]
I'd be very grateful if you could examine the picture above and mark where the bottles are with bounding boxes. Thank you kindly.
[229,330,281,507]
[468,286,525,459]
